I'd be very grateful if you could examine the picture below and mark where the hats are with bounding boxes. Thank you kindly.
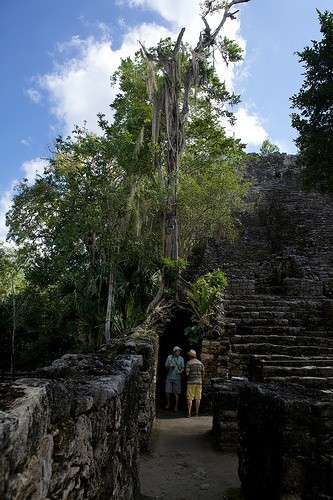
[187,349,197,357]
[172,346,182,352]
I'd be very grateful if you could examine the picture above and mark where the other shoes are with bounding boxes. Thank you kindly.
[166,406,170,409]
[194,414,199,417]
[174,407,178,412]
[185,414,191,417]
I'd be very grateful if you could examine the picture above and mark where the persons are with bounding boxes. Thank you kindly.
[165,347,185,414]
[186,349,205,418]
